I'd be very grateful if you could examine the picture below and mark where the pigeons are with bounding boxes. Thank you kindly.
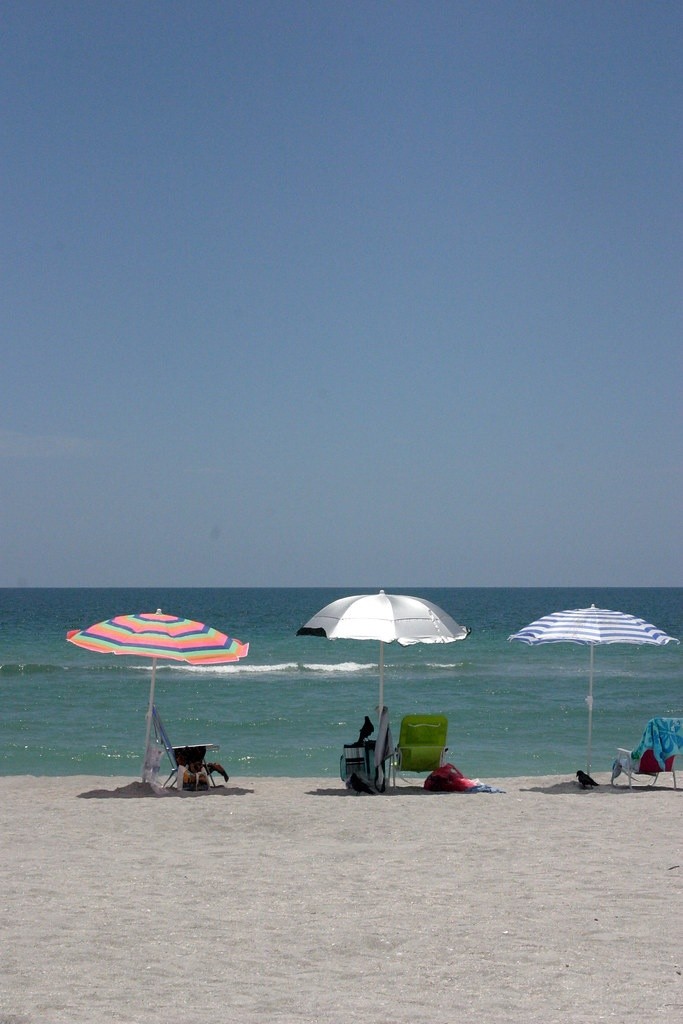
[576,770,599,789]
[358,716,374,742]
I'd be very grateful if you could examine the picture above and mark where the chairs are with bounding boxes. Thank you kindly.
[611,717,683,791]
[387,713,449,792]
[152,704,229,789]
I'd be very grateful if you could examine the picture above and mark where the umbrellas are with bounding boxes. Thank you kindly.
[66,608,249,783]
[296,590,471,785]
[508,604,680,776]
